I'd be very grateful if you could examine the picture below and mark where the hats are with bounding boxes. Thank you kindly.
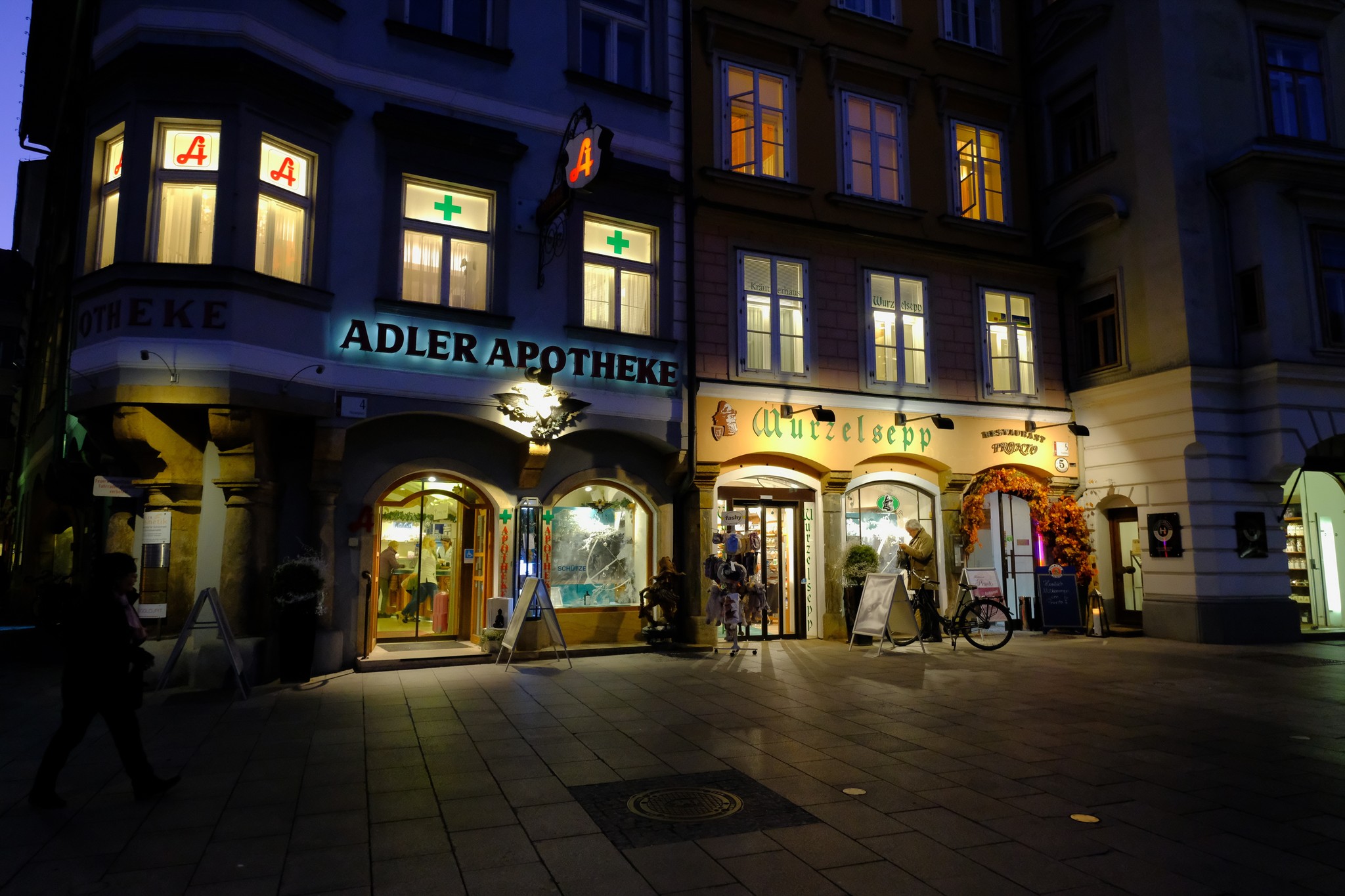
[441,536,451,541]
[90,552,136,579]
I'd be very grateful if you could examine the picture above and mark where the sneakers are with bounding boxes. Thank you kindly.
[395,611,404,625]
[378,612,392,618]
[407,617,421,623]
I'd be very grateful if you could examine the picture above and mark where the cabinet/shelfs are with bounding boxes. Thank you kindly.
[766,533,777,560]
[382,539,418,561]
[1280,517,1312,624]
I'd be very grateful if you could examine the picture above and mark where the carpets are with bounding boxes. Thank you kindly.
[1312,640,1345,646]
[1111,624,1143,629]
[376,640,474,652]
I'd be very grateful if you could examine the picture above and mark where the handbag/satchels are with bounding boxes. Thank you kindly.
[895,545,907,570]
[388,568,399,591]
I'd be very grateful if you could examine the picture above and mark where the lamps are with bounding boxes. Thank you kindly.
[1025,419,1090,437]
[781,404,836,423]
[279,364,326,394]
[140,350,179,383]
[895,412,955,430]
[524,365,554,386]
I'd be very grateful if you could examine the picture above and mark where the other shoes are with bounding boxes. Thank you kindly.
[27,788,67,809]
[132,776,182,804]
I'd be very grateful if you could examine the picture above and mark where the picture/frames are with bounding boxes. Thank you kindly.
[551,587,563,608]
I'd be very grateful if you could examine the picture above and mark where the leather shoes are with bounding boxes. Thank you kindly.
[915,632,931,641]
[922,634,942,642]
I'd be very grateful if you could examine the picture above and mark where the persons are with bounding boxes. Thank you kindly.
[27,552,182,806]
[493,609,504,628]
[396,534,444,626]
[899,519,943,643]
[376,540,406,618]
[437,535,452,569]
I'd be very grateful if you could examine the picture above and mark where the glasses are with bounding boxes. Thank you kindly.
[125,574,137,578]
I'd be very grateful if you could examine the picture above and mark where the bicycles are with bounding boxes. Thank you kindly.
[885,566,1014,651]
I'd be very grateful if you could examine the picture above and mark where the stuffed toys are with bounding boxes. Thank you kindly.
[705,583,768,640]
[720,559,747,587]
[712,532,721,544]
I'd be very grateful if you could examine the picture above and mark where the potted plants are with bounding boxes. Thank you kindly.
[271,561,327,683]
[842,543,880,646]
[478,629,505,653]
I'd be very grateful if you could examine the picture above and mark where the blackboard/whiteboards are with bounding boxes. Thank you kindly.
[1035,563,1083,628]
